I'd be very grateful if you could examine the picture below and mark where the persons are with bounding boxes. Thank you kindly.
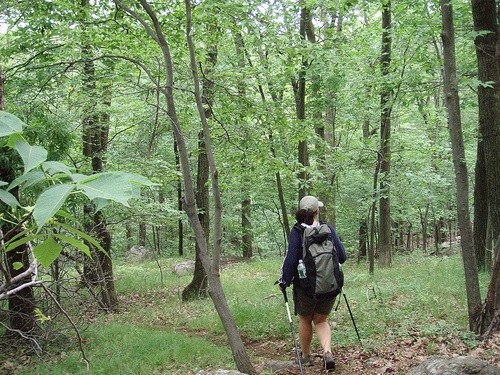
[278,196,347,372]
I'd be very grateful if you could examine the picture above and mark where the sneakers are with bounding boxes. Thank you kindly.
[323,351,336,372]
[295,352,314,367]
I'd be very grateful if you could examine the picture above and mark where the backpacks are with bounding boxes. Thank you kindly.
[293,221,344,299]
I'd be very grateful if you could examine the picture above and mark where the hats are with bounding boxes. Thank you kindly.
[300,196,324,212]
